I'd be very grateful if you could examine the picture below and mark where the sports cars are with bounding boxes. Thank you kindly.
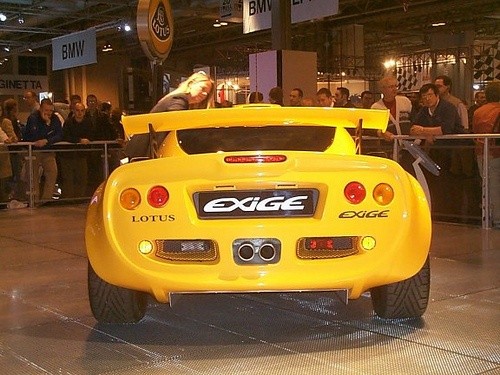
[84,102,431,323]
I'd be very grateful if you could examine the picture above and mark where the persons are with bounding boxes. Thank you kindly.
[0,126,12,209]
[433,75,469,131]
[23,90,41,112]
[49,76,483,226]
[23,102,64,207]
[467,90,487,134]
[111,70,215,170]
[472,79,500,229]
[0,98,29,209]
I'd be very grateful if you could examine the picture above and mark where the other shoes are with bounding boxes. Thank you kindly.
[10,200,28,209]
